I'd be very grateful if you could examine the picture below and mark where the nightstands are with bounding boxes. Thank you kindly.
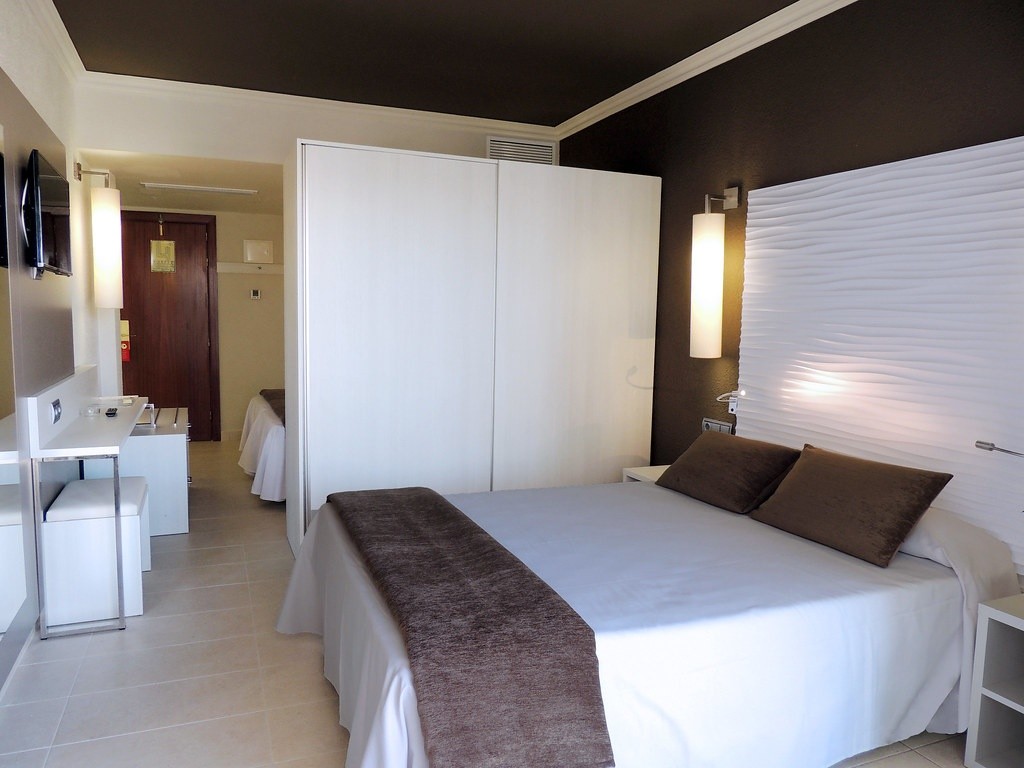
[963,594,1024,768]
[620,464,672,482]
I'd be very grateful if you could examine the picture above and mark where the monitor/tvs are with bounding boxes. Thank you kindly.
[24,149,73,278]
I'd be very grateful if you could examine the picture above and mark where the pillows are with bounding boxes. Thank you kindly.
[748,441,959,570]
[654,427,802,515]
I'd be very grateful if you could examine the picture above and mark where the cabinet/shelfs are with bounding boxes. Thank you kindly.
[80,408,192,538]
[278,132,668,555]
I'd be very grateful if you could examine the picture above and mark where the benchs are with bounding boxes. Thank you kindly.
[43,473,153,628]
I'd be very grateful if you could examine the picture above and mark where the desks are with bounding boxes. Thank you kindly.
[33,391,151,638]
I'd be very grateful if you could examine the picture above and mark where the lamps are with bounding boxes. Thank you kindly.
[716,389,748,415]
[72,161,124,311]
[688,185,743,358]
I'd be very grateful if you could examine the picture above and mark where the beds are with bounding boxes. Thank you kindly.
[271,474,1019,768]
[239,388,285,510]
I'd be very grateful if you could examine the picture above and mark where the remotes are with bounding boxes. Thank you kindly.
[106,407,118,417]
